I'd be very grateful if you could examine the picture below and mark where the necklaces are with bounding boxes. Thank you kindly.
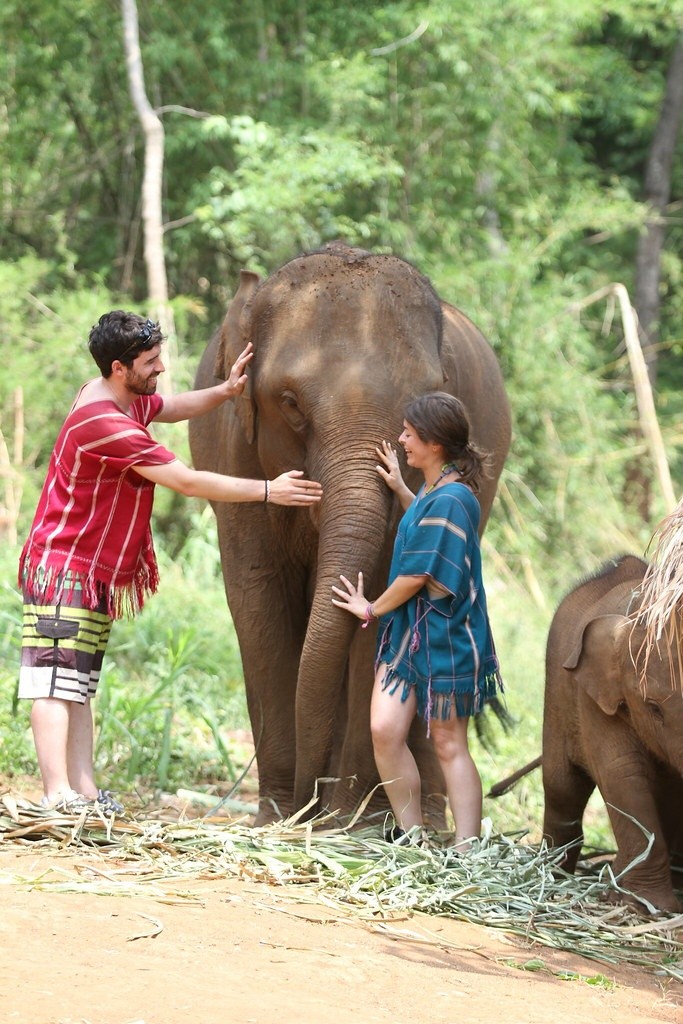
[424,464,457,496]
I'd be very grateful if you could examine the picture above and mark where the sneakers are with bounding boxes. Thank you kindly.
[382,824,426,848]
[42,789,125,815]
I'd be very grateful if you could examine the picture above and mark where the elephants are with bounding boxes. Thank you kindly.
[188,240,513,851]
[534,494,682,920]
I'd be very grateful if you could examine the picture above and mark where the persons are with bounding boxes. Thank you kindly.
[18,309,323,814]
[332,392,503,853]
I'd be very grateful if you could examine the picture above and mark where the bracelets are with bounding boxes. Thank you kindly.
[366,601,376,622]
[265,480,271,502]
